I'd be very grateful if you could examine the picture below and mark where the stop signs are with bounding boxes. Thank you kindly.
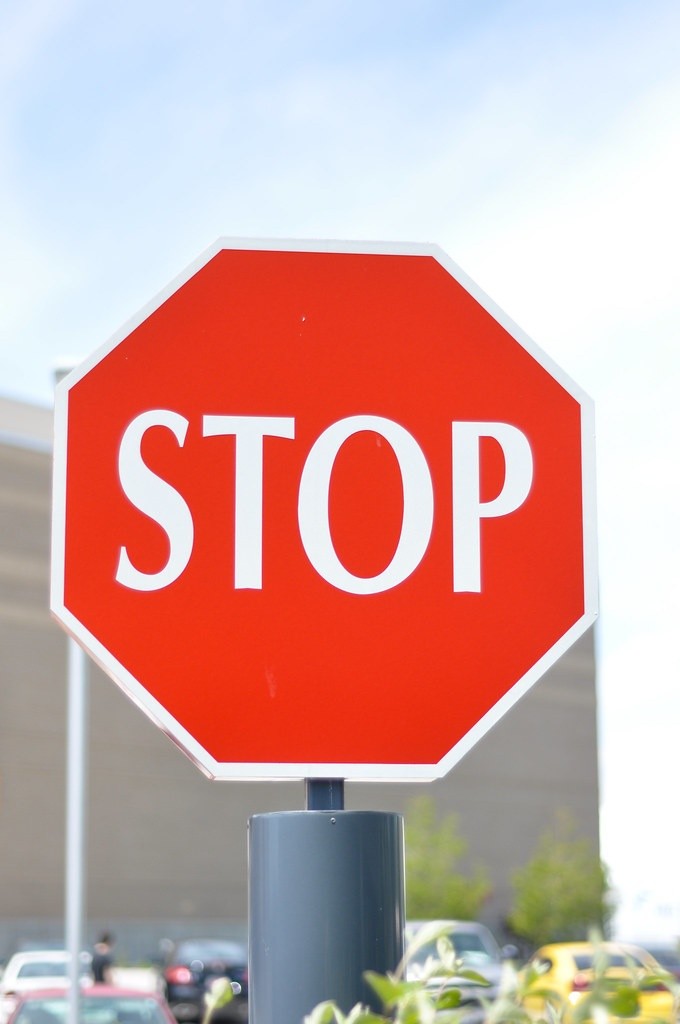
[57,245,585,767]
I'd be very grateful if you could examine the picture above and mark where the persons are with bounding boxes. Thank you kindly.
[90,933,115,986]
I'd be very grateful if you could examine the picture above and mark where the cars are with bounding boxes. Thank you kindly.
[405,921,521,1005]
[0,948,96,995]
[0,986,181,1024]
[104,964,168,1002]
[173,938,249,1024]
[518,939,680,1023]
